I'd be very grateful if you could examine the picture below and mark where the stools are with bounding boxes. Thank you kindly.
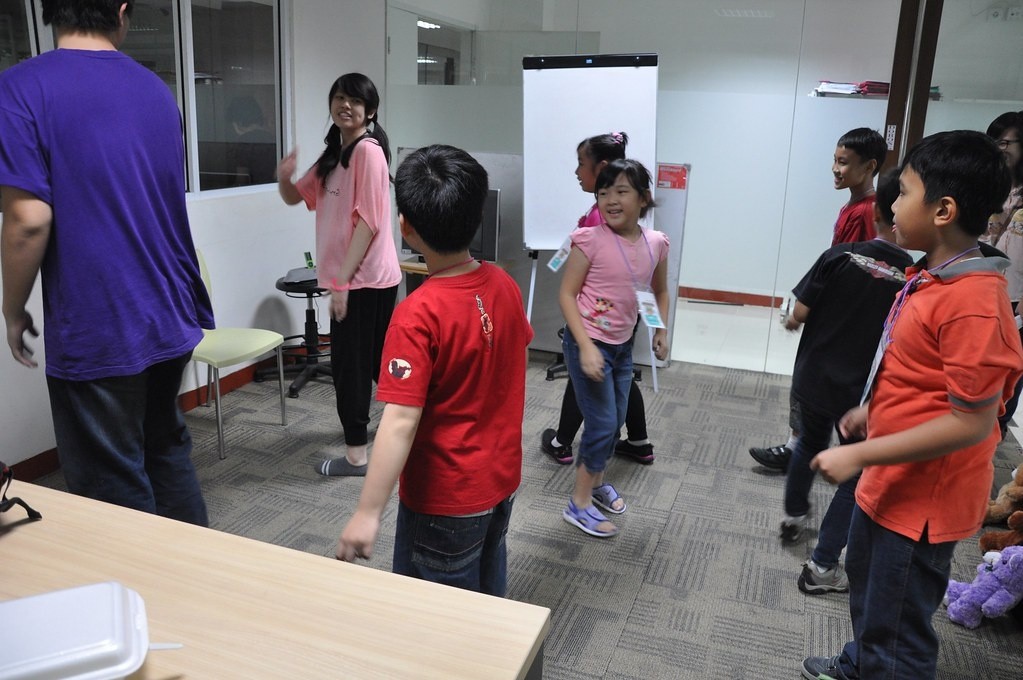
[258,266,352,396]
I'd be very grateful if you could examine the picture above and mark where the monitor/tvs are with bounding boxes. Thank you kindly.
[468,189,501,263]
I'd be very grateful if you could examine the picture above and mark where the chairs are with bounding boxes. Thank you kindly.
[166,250,298,466]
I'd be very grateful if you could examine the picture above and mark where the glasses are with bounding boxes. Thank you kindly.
[995,139,1020,150]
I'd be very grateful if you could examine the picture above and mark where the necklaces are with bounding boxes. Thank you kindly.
[427,257,473,281]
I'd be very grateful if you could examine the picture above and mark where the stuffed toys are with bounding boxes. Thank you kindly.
[944,463,1023,628]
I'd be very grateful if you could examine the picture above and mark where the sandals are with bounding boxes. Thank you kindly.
[563,497,619,537]
[592,482,626,514]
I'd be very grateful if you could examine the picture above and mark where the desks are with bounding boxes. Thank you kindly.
[397,253,515,309]
[0,468,551,680]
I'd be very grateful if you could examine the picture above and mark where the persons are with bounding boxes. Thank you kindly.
[222,98,277,186]
[748,112,1023,597]
[542,132,654,465]
[560,159,670,537]
[336,143,535,598]
[275,73,398,477]
[0,0,217,529]
[801,129,1023,680]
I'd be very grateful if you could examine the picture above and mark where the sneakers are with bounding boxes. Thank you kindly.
[614,439,655,464]
[749,444,794,471]
[541,429,574,464]
[780,508,814,541]
[798,561,850,595]
[800,655,850,680]
